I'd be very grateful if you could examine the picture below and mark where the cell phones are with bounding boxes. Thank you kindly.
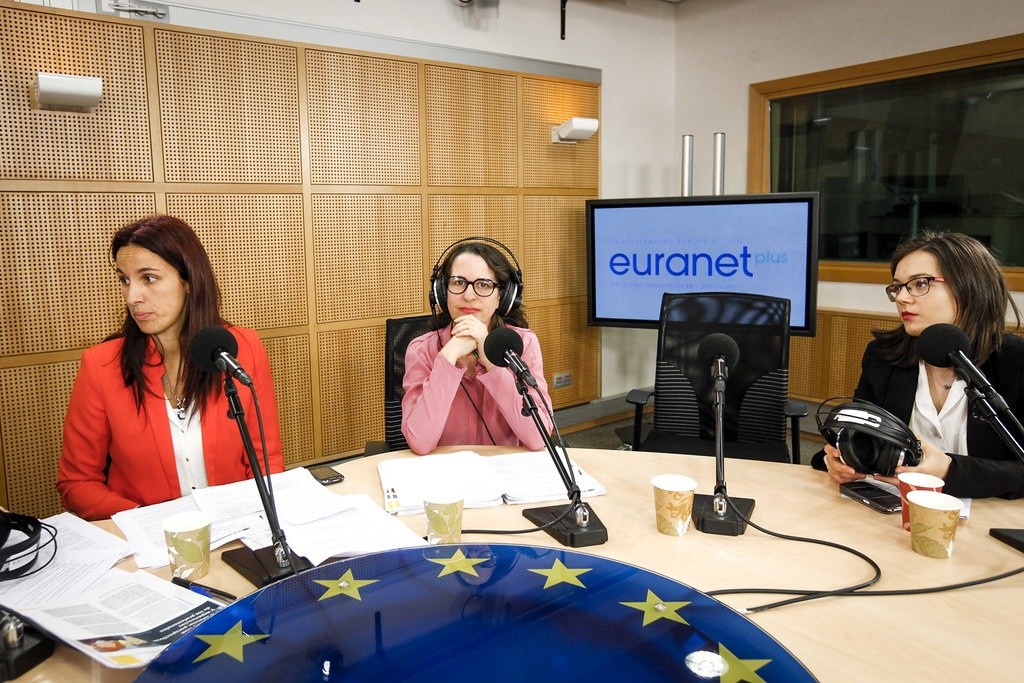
[839,481,903,514]
[308,466,345,484]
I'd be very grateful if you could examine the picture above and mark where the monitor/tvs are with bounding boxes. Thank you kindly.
[586,191,820,336]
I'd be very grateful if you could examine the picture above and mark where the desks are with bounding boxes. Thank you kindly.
[7,444,1024,683]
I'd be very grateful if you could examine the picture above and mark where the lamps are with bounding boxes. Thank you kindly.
[33,74,102,112]
[550,116,598,144]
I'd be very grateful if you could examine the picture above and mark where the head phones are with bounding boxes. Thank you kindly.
[428,237,524,317]
[815,396,925,477]
[0,510,57,582]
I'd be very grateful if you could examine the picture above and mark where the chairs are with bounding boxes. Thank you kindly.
[614,293,807,467]
[364,314,449,459]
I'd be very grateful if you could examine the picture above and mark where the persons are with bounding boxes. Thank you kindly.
[400,238,554,453]
[55,217,285,520]
[810,233,1024,499]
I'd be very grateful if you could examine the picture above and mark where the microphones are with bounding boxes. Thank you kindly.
[484,328,538,388]
[917,323,1010,412]
[698,333,740,392]
[189,326,253,387]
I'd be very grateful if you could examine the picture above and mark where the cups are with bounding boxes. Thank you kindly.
[422,488,465,546]
[652,473,697,536]
[906,491,964,558]
[898,473,945,532]
[163,510,212,580]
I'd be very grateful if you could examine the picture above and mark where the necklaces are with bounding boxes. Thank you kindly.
[162,374,188,420]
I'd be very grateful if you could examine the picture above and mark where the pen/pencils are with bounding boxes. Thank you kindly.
[172,577,236,607]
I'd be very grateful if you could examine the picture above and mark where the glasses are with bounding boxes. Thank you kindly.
[443,276,500,297]
[886,276,947,303]
